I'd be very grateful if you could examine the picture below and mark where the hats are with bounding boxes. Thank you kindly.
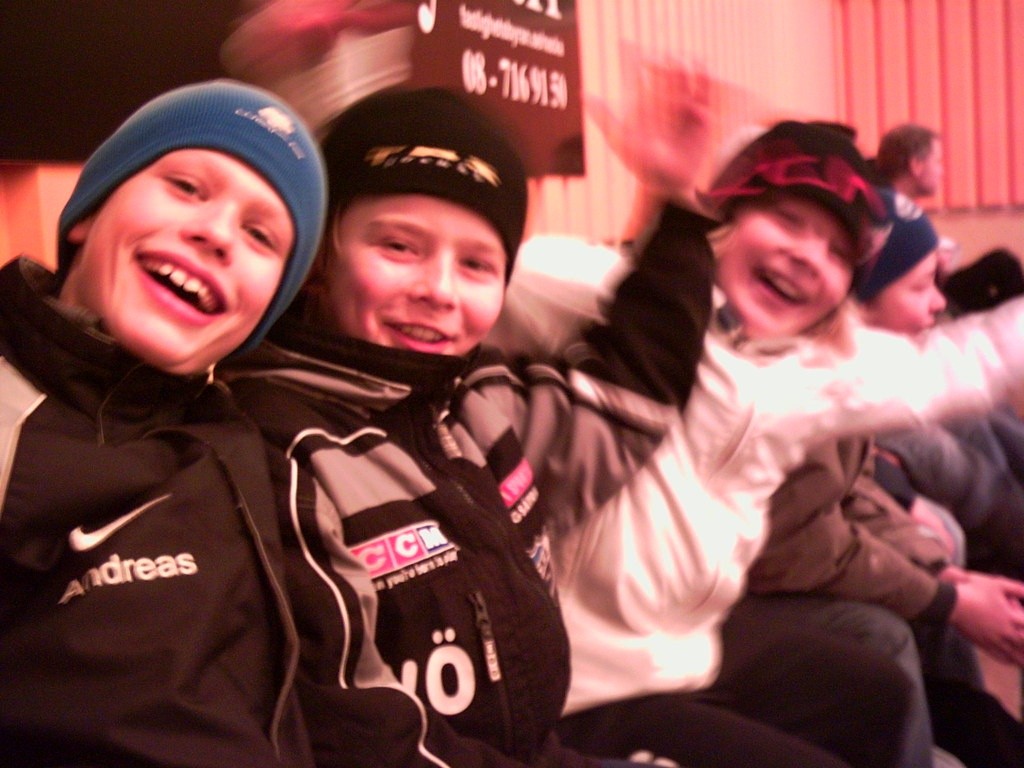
[695,118,888,262]
[848,188,939,302]
[322,87,528,287]
[57,79,327,355]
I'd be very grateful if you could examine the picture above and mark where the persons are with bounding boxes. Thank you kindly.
[484,119,1024,768]
[855,185,1024,768]
[0,78,327,768]
[219,51,715,768]
[873,124,945,200]
[746,435,1024,768]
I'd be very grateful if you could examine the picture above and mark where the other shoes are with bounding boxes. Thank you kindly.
[920,644,1024,768]
